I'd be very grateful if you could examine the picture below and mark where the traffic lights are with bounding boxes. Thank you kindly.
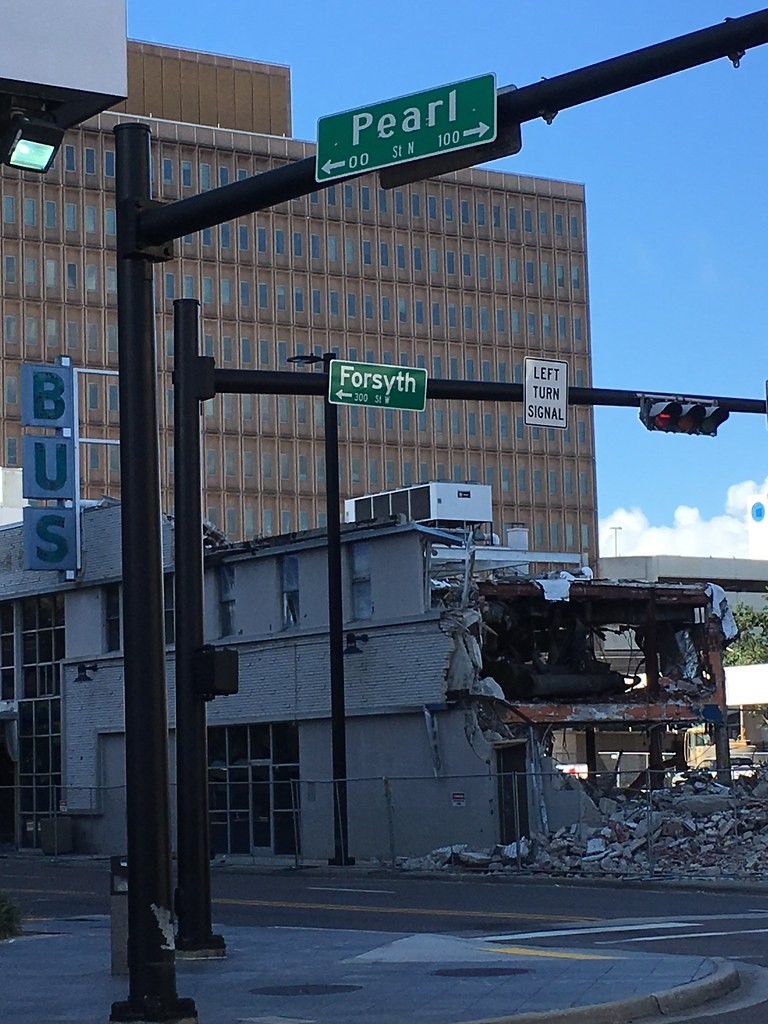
[198,649,240,696]
[640,398,730,437]
[314,70,497,184]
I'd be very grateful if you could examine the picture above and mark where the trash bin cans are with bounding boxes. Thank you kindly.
[108,853,130,976]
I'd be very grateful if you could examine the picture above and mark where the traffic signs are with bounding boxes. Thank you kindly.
[328,357,428,412]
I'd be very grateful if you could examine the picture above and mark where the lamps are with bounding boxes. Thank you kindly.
[342,632,370,656]
[3,108,63,174]
[72,664,97,683]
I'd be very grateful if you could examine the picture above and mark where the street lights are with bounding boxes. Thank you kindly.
[288,350,354,863]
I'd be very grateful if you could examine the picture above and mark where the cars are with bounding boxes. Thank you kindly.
[672,757,760,788]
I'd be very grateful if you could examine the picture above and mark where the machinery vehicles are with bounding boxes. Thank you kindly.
[684,725,758,768]
